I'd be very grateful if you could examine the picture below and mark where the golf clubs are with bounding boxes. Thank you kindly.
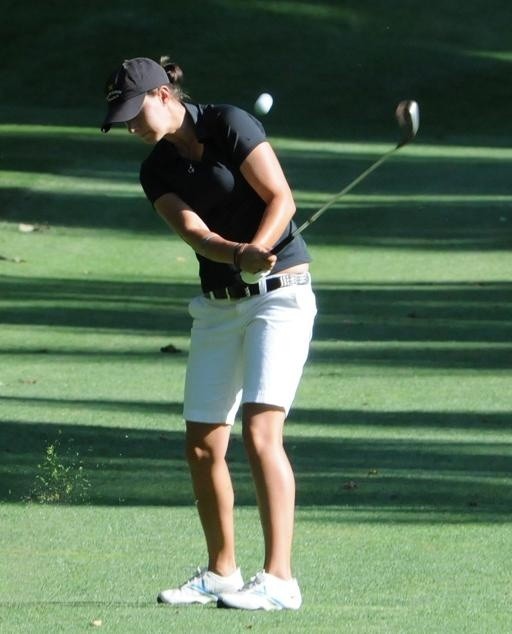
[269,99,419,253]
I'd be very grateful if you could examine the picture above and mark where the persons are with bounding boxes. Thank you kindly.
[101,55,318,611]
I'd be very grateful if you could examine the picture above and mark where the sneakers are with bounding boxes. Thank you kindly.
[217,568,302,610]
[157,564,244,605]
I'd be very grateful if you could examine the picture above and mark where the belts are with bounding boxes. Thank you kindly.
[203,273,309,301]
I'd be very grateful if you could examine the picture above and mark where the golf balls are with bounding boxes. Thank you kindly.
[253,92,273,115]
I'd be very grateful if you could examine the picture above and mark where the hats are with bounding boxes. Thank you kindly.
[101,57,170,134]
